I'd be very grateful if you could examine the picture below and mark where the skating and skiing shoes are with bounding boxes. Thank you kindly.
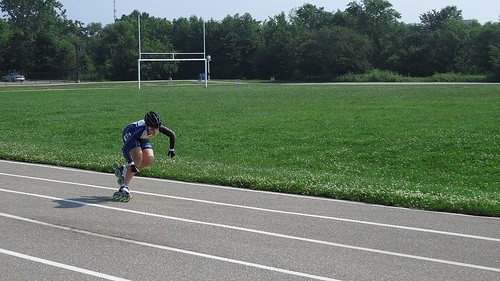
[113,161,127,185]
[113,186,131,202]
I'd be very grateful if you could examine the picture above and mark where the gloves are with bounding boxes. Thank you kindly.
[129,162,138,172]
[169,149,175,157]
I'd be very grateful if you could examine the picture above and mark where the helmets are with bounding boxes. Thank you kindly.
[145,111,161,128]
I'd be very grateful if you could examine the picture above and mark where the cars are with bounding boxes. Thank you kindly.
[1,73,25,82]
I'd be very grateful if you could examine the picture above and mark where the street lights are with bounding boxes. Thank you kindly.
[205,55,212,81]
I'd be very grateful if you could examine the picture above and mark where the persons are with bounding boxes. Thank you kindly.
[198,73,202,82]
[202,73,205,80]
[168,77,173,84]
[113,111,176,202]
